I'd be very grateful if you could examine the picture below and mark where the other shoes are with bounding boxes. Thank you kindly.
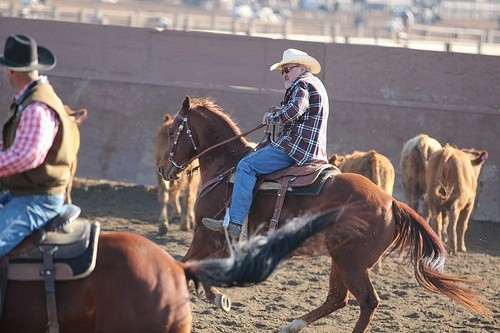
[202,218,241,238]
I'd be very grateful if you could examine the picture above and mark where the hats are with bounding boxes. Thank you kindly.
[270,49,321,74]
[0,33,57,72]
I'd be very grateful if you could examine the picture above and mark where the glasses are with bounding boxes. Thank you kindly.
[282,66,299,75]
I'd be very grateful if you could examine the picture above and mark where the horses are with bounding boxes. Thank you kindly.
[151,112,200,237]
[156,94,493,332]
[60,99,87,204]
[0,195,374,333]
[424,140,489,254]
[327,149,396,273]
[400,131,442,247]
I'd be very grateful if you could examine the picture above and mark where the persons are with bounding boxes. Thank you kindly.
[202,48,329,239]
[0,33,76,259]
